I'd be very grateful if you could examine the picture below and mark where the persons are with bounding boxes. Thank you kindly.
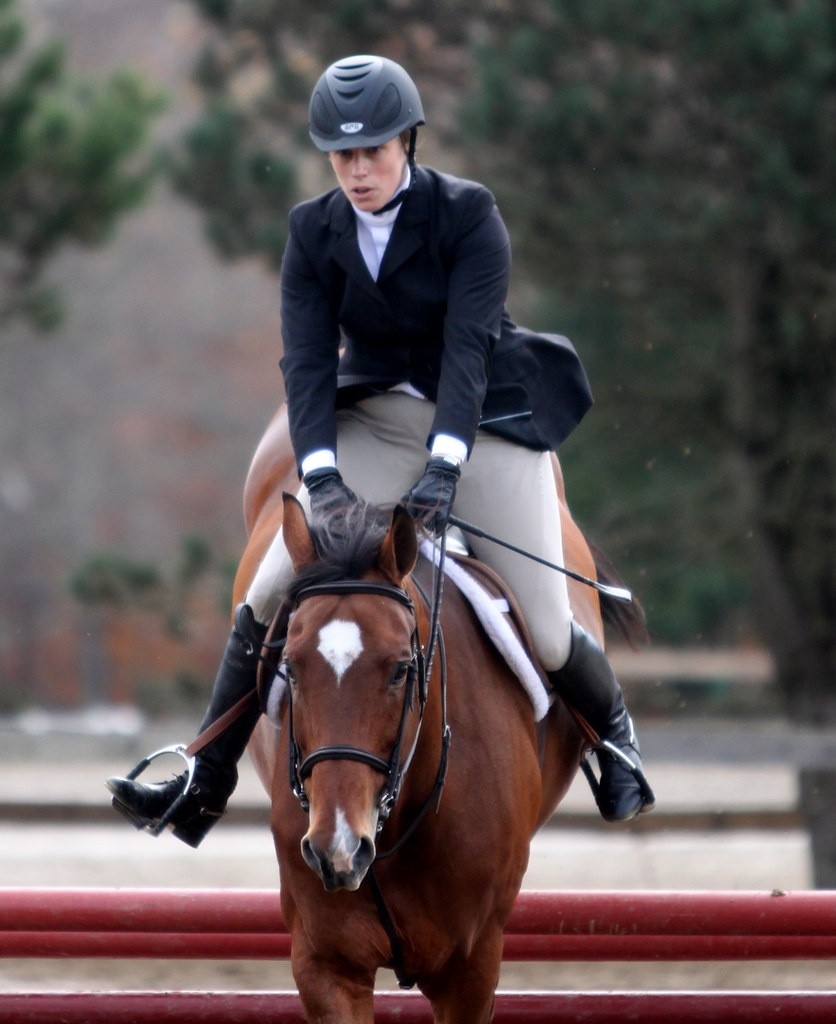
[104,54,650,850]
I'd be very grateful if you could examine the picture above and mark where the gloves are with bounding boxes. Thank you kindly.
[302,467,358,545]
[401,458,460,539]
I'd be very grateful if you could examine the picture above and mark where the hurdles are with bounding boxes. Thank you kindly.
[1,883,836,1024]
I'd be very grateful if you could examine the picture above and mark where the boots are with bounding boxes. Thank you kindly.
[541,619,643,823]
[106,616,281,850]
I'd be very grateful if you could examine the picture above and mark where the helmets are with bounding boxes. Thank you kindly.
[308,54,427,152]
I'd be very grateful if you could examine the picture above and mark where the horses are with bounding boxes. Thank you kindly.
[233,398,640,1016]
